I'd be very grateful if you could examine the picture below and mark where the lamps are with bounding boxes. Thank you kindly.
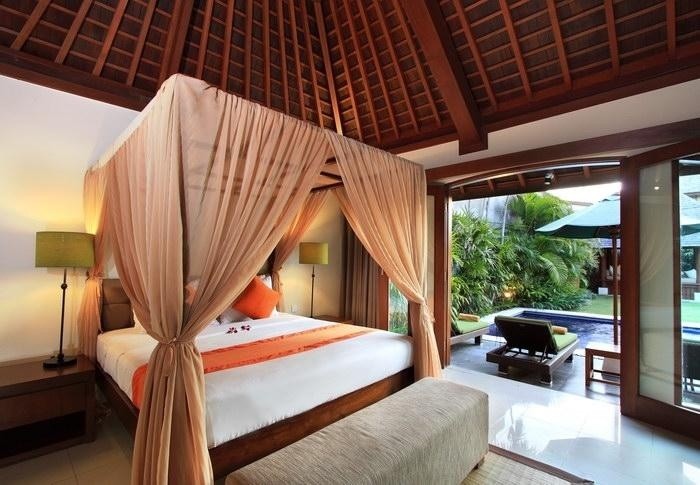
[35,231,95,371]
[298,242,328,320]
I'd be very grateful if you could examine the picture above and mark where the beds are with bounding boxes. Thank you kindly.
[92,275,418,485]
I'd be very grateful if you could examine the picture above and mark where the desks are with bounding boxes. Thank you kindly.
[584,342,621,388]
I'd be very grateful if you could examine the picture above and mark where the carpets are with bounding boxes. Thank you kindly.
[458,443,596,485]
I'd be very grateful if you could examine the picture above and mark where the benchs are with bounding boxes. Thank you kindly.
[224,375,491,485]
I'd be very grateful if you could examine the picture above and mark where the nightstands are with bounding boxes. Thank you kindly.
[0,355,100,469]
[314,315,356,325]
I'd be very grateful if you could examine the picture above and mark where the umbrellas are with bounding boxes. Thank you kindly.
[533,192,700,344]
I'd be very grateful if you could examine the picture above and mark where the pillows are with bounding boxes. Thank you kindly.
[458,313,480,322]
[231,275,283,319]
[552,325,568,335]
[186,271,266,305]
[133,303,219,332]
[192,276,277,324]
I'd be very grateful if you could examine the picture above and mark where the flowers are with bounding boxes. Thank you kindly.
[226,325,252,336]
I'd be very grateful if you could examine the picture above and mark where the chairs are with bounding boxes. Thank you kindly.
[485,315,581,385]
[682,338,699,396]
[449,312,490,350]
